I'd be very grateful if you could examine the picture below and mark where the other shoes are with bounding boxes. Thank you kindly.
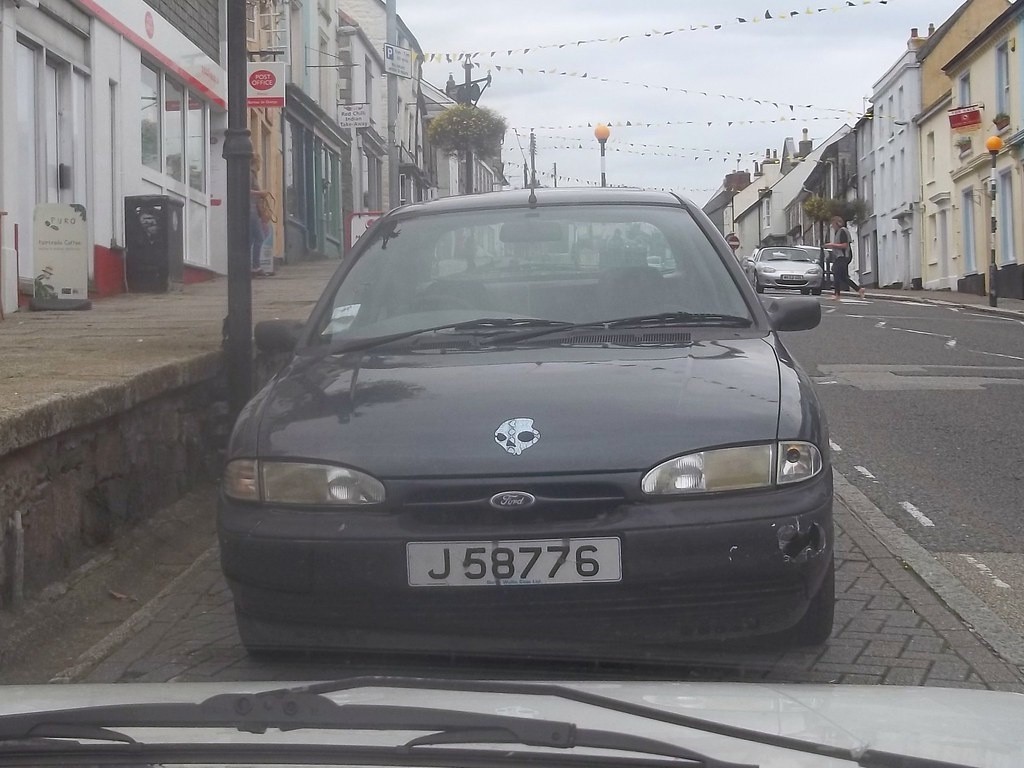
[859,288,865,300]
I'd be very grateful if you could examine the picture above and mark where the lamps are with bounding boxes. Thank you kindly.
[336,25,357,36]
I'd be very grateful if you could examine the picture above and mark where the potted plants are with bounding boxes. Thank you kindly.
[954,137,972,153]
[992,112,1010,131]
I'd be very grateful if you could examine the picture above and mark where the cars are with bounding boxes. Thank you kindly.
[215,186,835,650]
[745,245,835,289]
[746,247,825,295]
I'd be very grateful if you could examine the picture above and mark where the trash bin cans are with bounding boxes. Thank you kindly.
[123,194,185,285]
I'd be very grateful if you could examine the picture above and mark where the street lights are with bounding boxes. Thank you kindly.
[594,123,610,189]
[987,135,1003,308]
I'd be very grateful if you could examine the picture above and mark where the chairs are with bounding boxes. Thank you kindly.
[410,282,490,312]
[582,270,690,323]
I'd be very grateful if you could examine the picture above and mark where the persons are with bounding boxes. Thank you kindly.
[822,215,866,301]
[249,152,270,278]
